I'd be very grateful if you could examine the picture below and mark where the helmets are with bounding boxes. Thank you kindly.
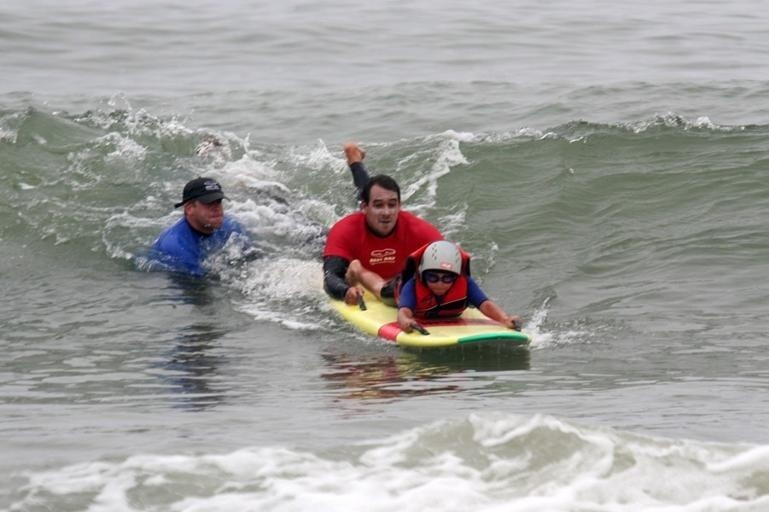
[419,240,463,282]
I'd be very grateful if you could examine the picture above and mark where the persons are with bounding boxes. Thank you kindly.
[344,240,521,334]
[139,178,249,276]
[323,142,471,306]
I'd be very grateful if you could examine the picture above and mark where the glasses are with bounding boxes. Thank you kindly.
[425,273,455,283]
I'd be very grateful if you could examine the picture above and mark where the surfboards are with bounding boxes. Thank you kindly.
[330,283,532,347]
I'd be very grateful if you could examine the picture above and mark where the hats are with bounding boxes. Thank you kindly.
[182,177,232,205]
